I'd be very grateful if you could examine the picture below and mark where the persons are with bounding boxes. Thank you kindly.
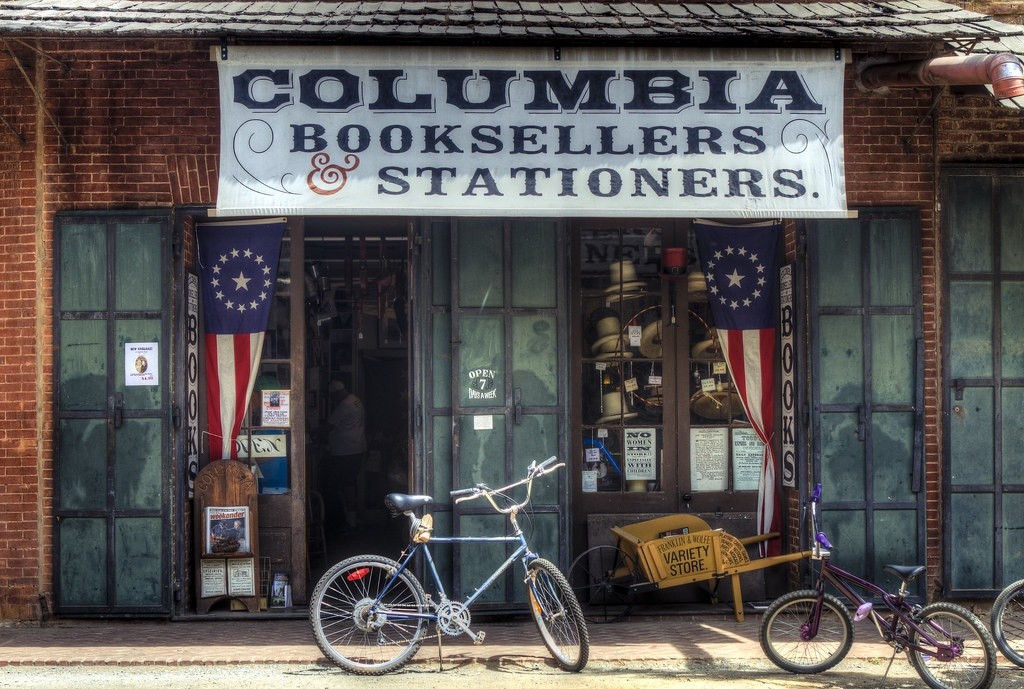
[311,381,368,533]
[208,519,246,551]
[135,357,147,372]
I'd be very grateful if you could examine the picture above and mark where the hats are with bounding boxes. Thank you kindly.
[604,260,648,304]
[644,385,662,414]
[592,317,632,367]
[639,319,662,359]
[687,272,708,301]
[691,382,745,421]
[692,327,726,364]
[595,391,638,424]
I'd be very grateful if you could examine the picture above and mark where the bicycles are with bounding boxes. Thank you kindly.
[308,456,590,676]
[757,483,1024,689]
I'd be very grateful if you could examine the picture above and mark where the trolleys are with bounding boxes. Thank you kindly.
[605,513,828,621]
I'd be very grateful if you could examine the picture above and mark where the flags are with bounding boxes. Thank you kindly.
[196,217,284,463]
[693,218,787,561]
